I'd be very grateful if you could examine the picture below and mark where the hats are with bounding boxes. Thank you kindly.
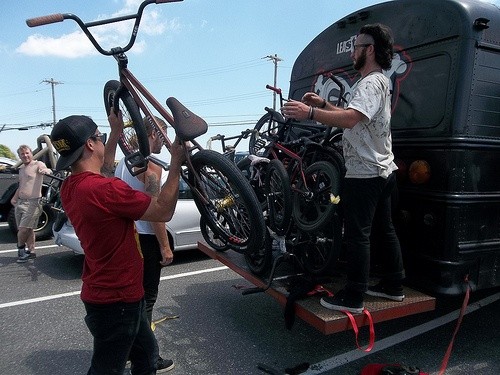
[50,115,98,171]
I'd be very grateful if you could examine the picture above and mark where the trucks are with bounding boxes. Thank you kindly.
[198,0,500,335]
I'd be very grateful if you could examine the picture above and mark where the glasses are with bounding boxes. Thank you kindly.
[90,133,107,144]
[352,43,372,50]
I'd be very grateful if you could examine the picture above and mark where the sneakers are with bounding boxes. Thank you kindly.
[17,243,26,257]
[129,356,174,375]
[320,295,363,313]
[364,285,404,302]
[17,251,36,262]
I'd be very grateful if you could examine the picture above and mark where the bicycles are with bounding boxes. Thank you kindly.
[199,70,353,297]
[44,164,71,232]
[25,0,267,255]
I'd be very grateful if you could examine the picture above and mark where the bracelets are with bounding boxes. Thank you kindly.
[320,99,327,108]
[308,106,313,119]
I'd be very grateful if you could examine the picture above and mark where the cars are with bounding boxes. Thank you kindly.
[52,148,262,270]
[0,157,62,242]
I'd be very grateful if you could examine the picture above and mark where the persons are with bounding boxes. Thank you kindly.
[50,108,194,375]
[10,145,53,263]
[281,25,407,311]
